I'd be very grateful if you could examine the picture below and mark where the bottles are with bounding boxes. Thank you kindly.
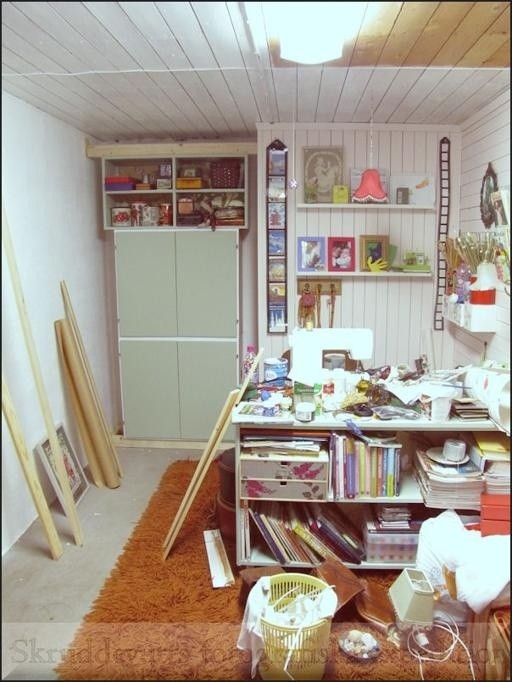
[241,345,259,385]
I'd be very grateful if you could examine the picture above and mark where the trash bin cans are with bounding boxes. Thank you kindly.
[247,574,337,682]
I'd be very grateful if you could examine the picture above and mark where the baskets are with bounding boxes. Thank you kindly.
[210,161,241,188]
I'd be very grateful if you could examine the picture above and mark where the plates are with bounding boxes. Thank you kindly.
[426,446,470,465]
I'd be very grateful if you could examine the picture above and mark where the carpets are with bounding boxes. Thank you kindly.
[53,453,476,682]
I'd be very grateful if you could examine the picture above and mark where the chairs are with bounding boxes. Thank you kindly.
[291,326,373,392]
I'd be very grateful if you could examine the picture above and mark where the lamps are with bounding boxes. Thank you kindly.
[351,109,391,203]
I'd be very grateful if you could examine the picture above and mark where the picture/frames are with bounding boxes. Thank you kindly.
[265,138,288,335]
[297,234,392,272]
[301,144,343,203]
[36,426,91,511]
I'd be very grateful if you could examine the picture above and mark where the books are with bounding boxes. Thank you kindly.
[236,420,511,573]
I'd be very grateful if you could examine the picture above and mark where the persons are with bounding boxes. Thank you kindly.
[301,240,352,270]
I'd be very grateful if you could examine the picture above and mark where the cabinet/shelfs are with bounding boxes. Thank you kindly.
[296,202,435,279]
[97,152,252,445]
[227,400,499,571]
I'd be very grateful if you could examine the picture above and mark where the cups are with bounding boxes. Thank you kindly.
[109,199,173,225]
